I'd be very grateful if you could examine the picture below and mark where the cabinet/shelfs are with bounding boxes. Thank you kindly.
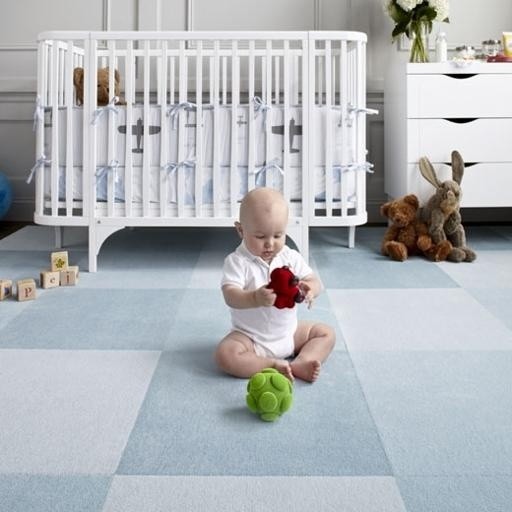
[382,64,512,222]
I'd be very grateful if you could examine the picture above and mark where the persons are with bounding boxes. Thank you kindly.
[216,187,335,385]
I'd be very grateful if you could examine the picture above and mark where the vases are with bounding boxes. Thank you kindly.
[408,20,429,62]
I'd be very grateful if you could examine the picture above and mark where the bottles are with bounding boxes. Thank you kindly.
[434,31,449,63]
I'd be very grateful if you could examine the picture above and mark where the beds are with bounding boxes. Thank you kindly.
[32,28,369,273]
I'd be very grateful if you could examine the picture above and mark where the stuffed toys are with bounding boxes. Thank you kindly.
[419,150,476,263]
[379,194,451,263]
[73,66,126,104]
[264,265,305,311]
[245,367,293,422]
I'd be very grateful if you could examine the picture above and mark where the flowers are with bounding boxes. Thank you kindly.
[381,0,450,62]
